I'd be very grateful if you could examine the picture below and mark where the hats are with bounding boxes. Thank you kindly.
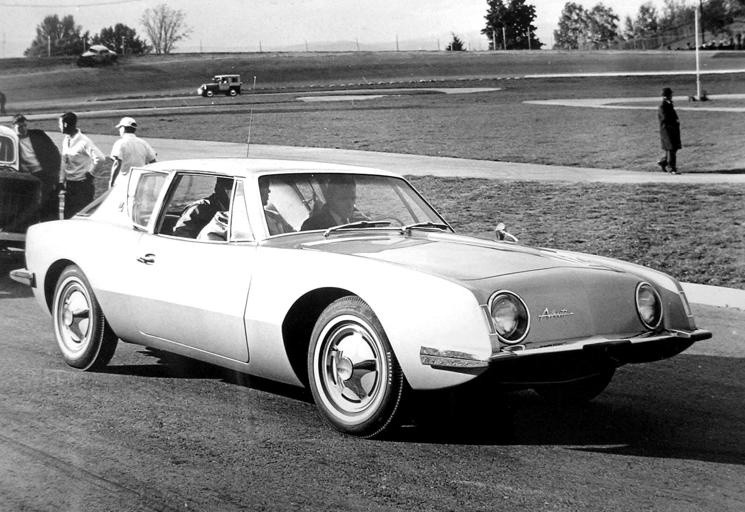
[660,88,674,97]
[113,116,137,129]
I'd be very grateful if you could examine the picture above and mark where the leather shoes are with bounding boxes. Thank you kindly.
[656,160,682,174]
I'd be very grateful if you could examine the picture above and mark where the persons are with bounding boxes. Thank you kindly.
[170,178,233,239]
[299,176,358,232]
[12,114,61,222]
[108,114,159,188]
[195,178,298,244]
[58,110,107,219]
[655,84,684,174]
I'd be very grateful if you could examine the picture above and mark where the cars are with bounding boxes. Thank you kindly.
[21,152,715,442]
[81,44,118,64]
[1,125,45,251]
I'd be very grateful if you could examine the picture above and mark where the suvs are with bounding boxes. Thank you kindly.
[201,75,241,97]
